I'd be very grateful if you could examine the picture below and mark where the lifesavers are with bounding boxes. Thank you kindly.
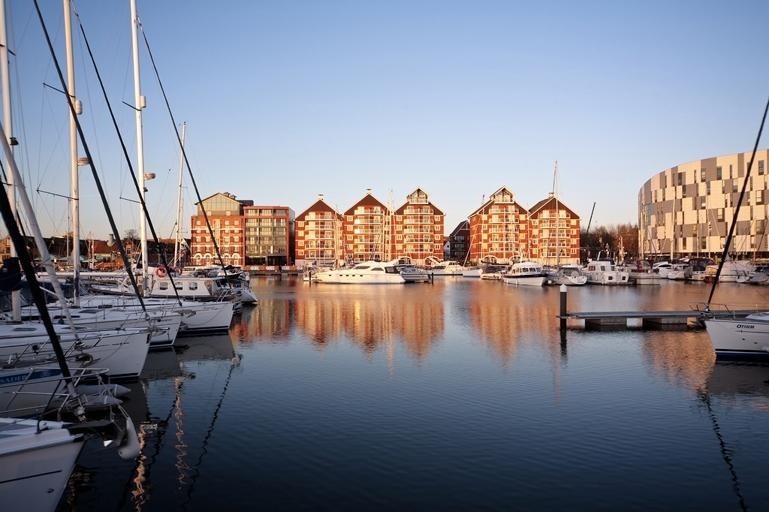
[156,267,167,278]
[174,267,183,275]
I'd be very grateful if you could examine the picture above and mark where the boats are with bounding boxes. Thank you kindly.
[432,259,768,287]
[304,260,430,284]
[705,310,768,365]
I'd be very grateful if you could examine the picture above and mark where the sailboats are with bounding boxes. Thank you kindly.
[0,0,260,464]
[0,124,118,511]
